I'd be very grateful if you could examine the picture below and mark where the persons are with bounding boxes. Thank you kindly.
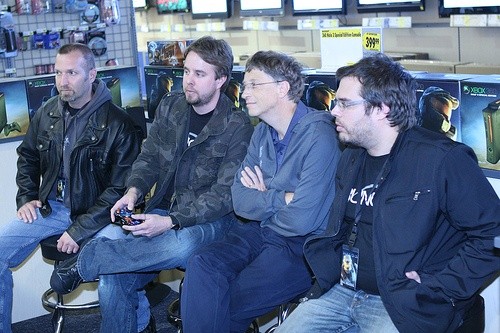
[0,44,157,333]
[419,86,459,139]
[50,36,254,333]
[149,71,173,113]
[273,53,500,333]
[224,77,242,107]
[181,51,340,333]
[306,80,336,111]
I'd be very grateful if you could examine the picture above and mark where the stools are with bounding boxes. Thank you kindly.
[165,266,186,333]
[251,283,310,333]
[458,294,485,333]
[38,235,100,333]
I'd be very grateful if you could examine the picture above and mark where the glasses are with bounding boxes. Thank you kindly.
[246,81,281,90]
[334,99,367,112]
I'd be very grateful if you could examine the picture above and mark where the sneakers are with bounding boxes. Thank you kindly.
[50,238,97,295]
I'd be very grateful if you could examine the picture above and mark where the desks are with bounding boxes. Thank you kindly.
[145,64,500,178]
[0,64,142,142]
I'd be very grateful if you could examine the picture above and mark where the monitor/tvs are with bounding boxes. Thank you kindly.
[131,0,500,18]
